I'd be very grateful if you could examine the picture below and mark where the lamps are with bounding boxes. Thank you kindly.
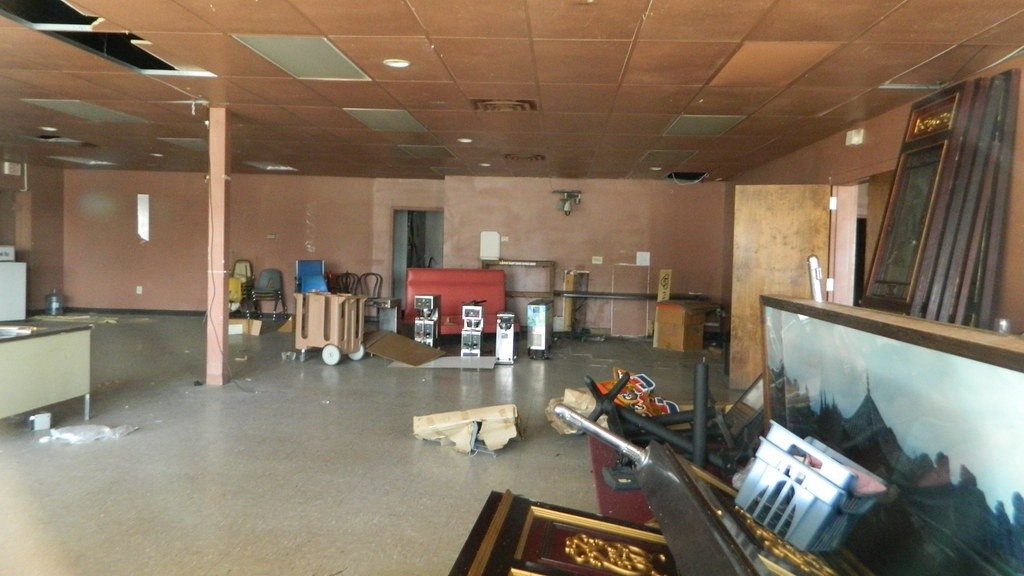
[551,190,582,217]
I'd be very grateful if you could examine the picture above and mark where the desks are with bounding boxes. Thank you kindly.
[0,321,92,422]
[556,291,707,301]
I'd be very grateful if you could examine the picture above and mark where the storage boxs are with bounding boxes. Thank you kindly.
[732,419,890,553]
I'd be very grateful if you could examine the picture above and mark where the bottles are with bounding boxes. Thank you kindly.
[45,288,63,315]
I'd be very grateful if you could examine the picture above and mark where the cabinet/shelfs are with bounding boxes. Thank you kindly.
[483,260,557,332]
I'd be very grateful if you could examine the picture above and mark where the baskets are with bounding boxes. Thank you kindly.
[736,420,887,552]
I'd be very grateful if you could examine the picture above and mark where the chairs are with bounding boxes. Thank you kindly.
[359,273,383,331]
[336,270,360,294]
[229,258,289,322]
[294,258,329,293]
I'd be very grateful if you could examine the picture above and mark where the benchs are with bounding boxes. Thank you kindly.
[405,267,521,341]
